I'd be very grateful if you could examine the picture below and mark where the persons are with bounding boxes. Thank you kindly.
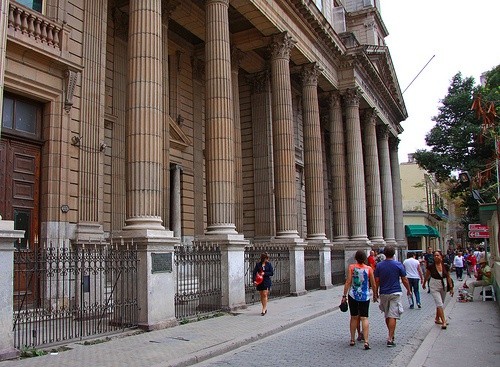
[341,250,378,348]
[422,251,454,328]
[403,252,423,308]
[252,253,273,315]
[363,245,495,302]
[375,246,412,347]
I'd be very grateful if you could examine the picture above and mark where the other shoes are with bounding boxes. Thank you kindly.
[364,342,371,349]
[357,336,362,340]
[350,340,356,345]
[410,305,414,309]
[435,320,443,324]
[387,341,396,347]
[442,325,446,328]
[418,304,421,308]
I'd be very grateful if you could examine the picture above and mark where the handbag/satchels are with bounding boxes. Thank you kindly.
[254,267,263,285]
[443,264,454,292]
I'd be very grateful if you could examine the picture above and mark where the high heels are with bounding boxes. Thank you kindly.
[261,309,267,314]
[262,312,265,316]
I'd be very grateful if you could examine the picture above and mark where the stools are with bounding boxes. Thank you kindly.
[482,285,496,302]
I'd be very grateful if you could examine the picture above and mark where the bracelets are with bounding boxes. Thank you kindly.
[343,295,347,297]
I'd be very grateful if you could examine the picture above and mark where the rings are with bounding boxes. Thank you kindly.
[342,299,343,301]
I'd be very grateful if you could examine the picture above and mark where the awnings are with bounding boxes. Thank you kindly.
[404,224,441,239]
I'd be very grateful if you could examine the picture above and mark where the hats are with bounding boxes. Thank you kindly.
[479,258,487,263]
